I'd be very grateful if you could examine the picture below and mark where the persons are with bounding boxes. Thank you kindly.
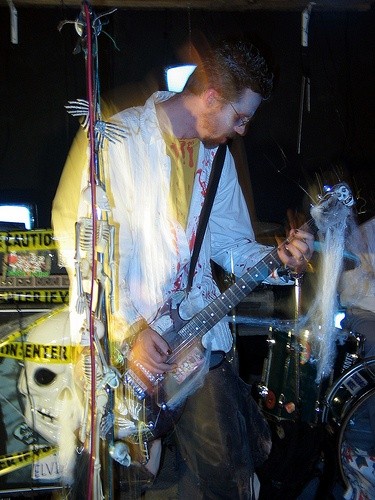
[51,42,315,500]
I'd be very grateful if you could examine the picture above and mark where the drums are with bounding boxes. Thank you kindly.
[317,355,375,500]
[254,320,367,426]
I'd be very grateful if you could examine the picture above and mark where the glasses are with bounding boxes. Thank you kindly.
[229,100,252,129]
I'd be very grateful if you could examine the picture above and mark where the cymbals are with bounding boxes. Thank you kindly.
[251,218,361,275]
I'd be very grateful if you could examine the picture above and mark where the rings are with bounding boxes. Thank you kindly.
[298,255,303,260]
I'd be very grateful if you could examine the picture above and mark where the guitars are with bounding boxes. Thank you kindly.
[107,181,358,470]
